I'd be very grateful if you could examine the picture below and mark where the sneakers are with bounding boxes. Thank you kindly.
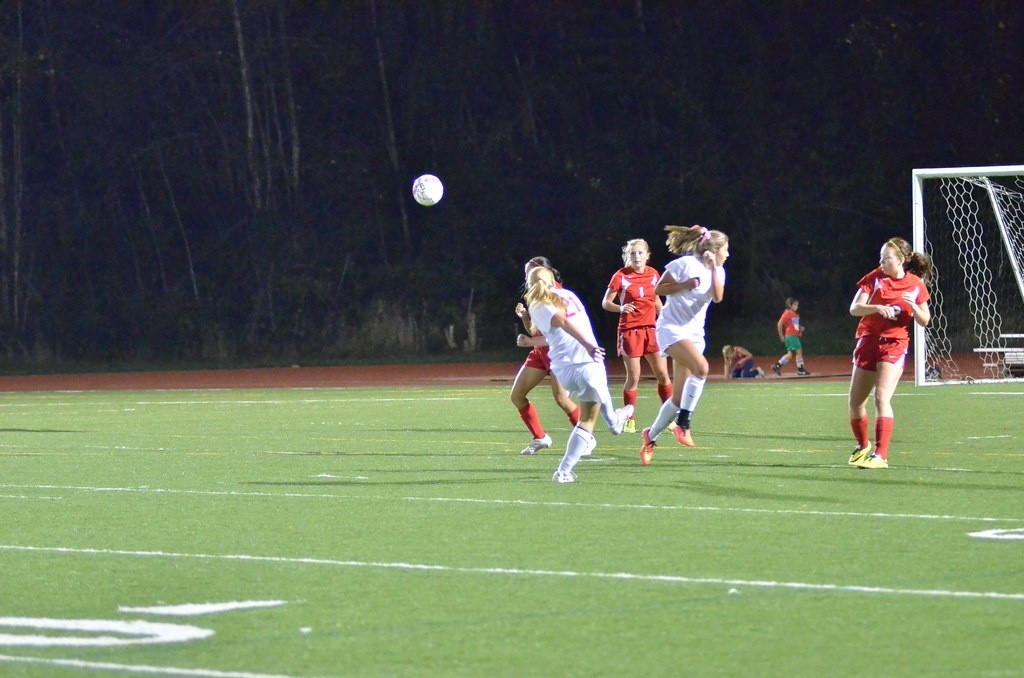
[667,421,676,431]
[858,453,888,469]
[521,433,552,455]
[673,425,696,447]
[623,419,636,433]
[640,427,658,464]
[849,440,871,465]
[581,435,597,455]
[609,404,634,435]
[552,471,577,483]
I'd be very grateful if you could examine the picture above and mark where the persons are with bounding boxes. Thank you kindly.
[601,239,673,433]
[525,266,634,483]
[640,225,729,465]
[771,297,810,376]
[510,257,580,455]
[722,344,765,379]
[848,237,931,469]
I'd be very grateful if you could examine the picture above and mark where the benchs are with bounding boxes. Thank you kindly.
[973,333,1024,367]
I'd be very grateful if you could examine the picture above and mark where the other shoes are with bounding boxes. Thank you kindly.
[797,364,810,375]
[756,366,765,376]
[772,363,783,376]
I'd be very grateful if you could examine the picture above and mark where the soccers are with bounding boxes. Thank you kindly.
[412,175,443,205]
